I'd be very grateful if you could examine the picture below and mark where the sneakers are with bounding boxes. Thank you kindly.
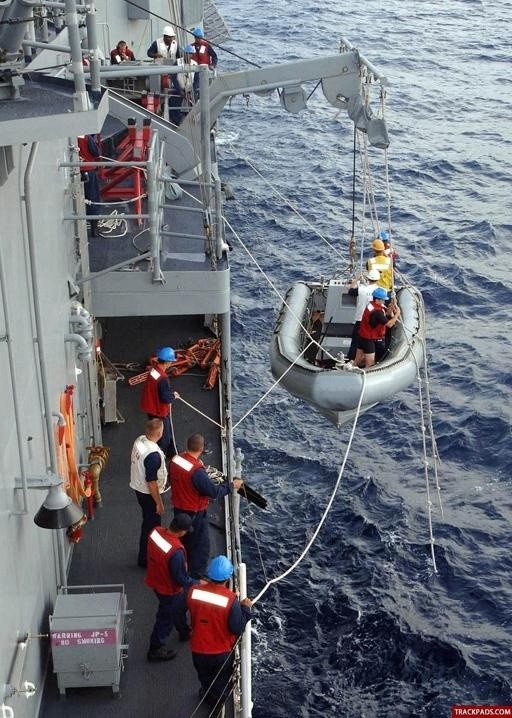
[148,647,176,661]
[179,627,193,640]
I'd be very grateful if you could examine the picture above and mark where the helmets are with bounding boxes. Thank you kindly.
[209,555,233,581]
[158,347,176,362]
[366,270,380,280]
[371,233,388,250]
[372,288,389,300]
[163,26,176,37]
[193,28,204,36]
[184,45,195,53]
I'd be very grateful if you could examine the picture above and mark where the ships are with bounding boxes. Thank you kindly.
[0,1,394,718]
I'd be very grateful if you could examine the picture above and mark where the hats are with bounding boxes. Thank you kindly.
[176,514,193,532]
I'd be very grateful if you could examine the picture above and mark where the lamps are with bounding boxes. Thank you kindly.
[14,471,84,529]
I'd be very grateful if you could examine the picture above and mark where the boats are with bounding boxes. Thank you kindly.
[269,280,426,427]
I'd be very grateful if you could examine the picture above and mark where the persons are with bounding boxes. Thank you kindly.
[146,26,182,116]
[139,512,200,666]
[141,345,182,460]
[74,131,104,239]
[164,432,243,583]
[181,28,219,107]
[345,228,401,370]
[167,42,201,125]
[127,416,171,574]
[185,552,253,718]
[106,40,139,95]
